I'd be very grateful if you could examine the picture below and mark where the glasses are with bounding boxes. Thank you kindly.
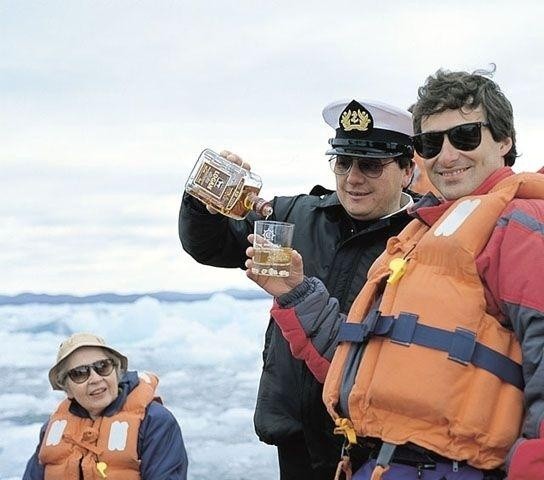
[329,155,400,178]
[409,122,490,159]
[61,358,118,383]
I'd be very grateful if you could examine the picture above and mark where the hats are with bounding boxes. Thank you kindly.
[322,99,415,158]
[49,333,128,390]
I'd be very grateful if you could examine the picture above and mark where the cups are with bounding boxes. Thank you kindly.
[250,220,295,278]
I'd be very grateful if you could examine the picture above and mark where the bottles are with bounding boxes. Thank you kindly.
[184,149,274,221]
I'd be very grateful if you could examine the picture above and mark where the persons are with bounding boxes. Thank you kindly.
[23,333,189,479]
[244,65,543,479]
[180,101,430,479]
